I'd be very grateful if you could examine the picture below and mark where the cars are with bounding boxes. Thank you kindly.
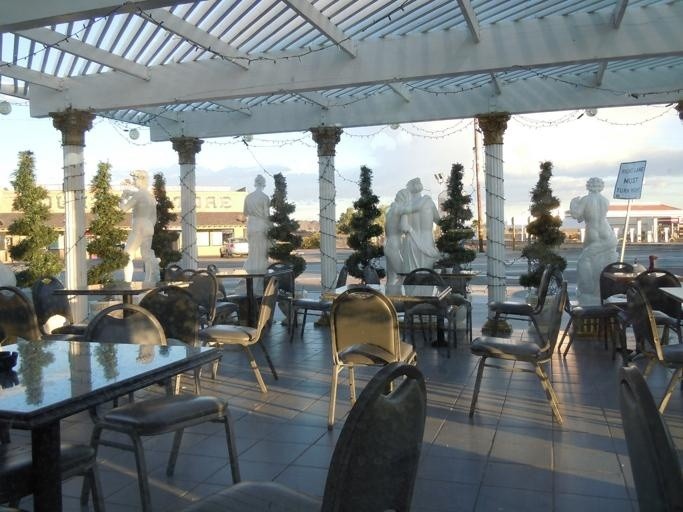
[217,235,252,258]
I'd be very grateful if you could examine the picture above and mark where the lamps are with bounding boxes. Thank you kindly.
[585,108,598,116]
[391,123,399,129]
[130,129,139,139]
[245,134,253,142]
[0,101,12,114]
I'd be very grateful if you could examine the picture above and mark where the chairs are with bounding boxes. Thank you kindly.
[470,282,568,424]
[618,366,683,512]
[116,286,199,403]
[638,269,682,356]
[80,303,241,511]
[195,274,279,396]
[263,262,298,334]
[329,285,419,430]
[31,276,88,341]
[488,263,552,347]
[0,286,40,444]
[168,265,182,275]
[600,262,634,363]
[180,362,427,512]
[177,269,196,282]
[207,263,240,326]
[185,270,218,347]
[626,282,683,414]
[429,270,473,345]
[558,281,623,361]
[290,264,347,342]
[404,268,452,348]
[0,325,106,512]
[364,265,380,285]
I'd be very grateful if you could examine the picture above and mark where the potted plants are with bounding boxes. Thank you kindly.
[9,149,65,302]
[433,163,478,299]
[519,161,566,334]
[150,172,183,280]
[86,159,131,301]
[266,172,308,325]
